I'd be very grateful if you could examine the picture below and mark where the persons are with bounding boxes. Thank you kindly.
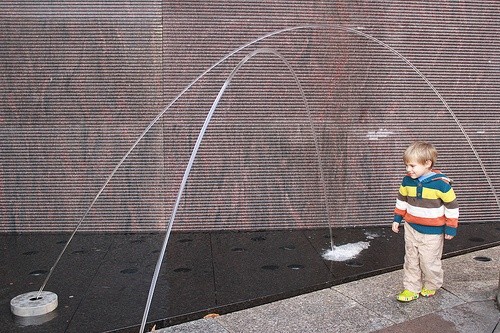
[392,142,460,302]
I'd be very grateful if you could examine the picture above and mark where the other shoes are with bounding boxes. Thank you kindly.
[397,289,420,302]
[421,287,436,297]
